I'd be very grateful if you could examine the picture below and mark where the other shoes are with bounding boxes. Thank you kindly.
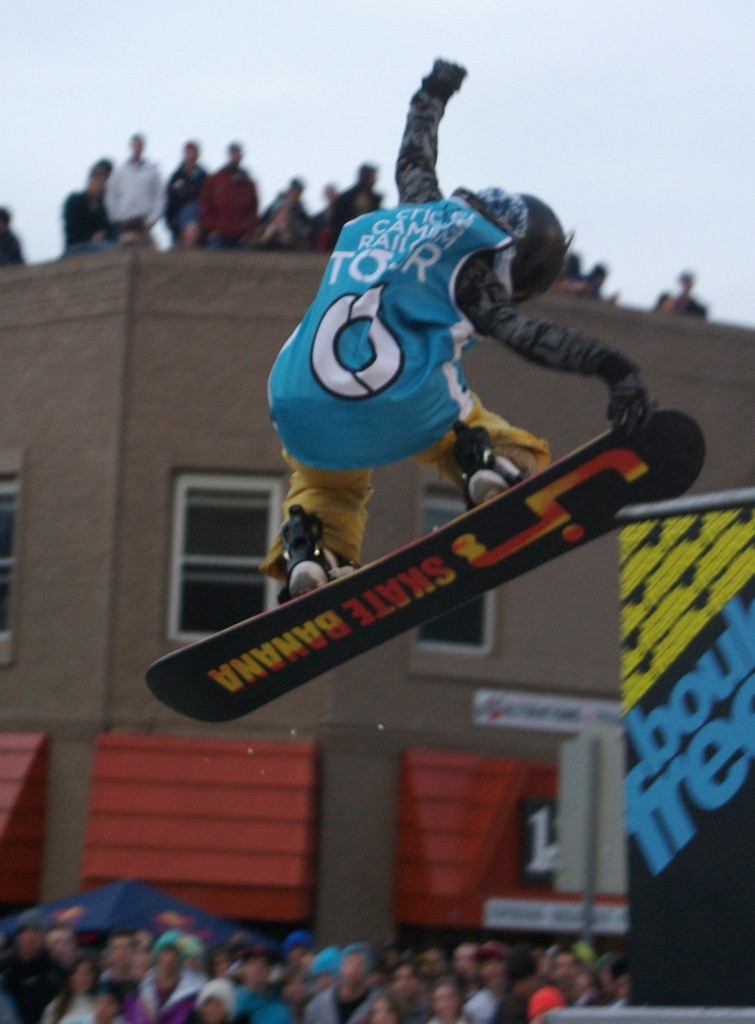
[463,461,508,505]
[283,547,328,598]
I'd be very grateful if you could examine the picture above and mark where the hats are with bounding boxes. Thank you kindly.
[342,942,376,964]
[283,930,314,954]
[473,940,510,961]
[195,977,235,1015]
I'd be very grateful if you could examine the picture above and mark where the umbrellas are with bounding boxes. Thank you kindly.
[0,880,281,952]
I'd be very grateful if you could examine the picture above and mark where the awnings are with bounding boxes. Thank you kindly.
[79,731,324,924]
[391,748,559,931]
[0,732,49,907]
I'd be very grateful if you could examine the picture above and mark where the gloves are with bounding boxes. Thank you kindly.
[426,59,468,93]
[605,373,661,437]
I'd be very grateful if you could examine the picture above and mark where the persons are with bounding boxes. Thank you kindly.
[256,58,655,600]
[0,134,384,268]
[653,267,711,319]
[564,249,622,303]
[0,911,629,1024]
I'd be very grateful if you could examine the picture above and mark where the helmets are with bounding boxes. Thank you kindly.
[512,189,570,301]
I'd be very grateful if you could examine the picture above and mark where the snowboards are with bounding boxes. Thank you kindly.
[144,406,706,723]
